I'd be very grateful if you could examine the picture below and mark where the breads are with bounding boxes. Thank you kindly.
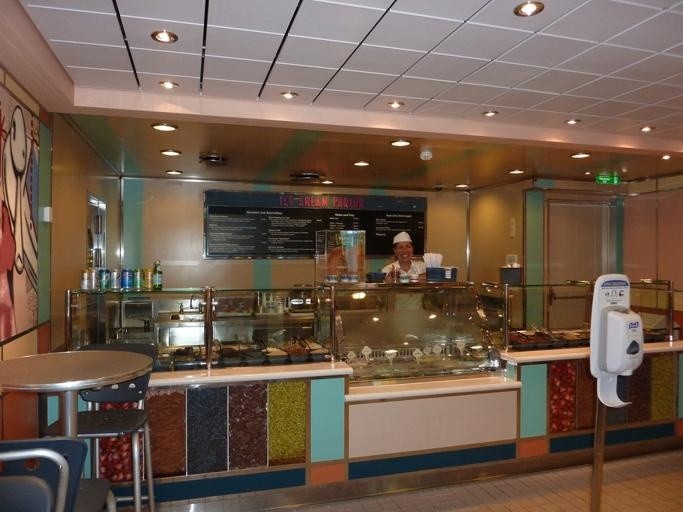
[170,347,193,362]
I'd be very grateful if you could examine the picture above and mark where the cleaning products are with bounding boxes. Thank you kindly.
[255,292,278,314]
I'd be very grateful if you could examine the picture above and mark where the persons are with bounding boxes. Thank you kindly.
[379,230,435,343]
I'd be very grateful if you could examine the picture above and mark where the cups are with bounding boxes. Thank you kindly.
[410,274,419,283]
[387,264,398,285]
[397,274,408,283]
[325,272,359,282]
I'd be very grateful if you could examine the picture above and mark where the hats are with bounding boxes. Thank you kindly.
[393,230,412,243]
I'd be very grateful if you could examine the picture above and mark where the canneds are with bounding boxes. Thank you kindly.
[89,248,101,269]
[80,268,154,291]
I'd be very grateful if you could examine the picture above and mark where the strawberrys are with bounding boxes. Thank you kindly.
[98,402,143,482]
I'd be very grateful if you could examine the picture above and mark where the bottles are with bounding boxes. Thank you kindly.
[151,260,162,291]
[254,291,283,315]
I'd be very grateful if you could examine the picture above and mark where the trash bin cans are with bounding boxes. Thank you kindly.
[638,312,681,341]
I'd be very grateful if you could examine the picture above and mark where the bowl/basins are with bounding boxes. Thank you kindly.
[366,272,385,282]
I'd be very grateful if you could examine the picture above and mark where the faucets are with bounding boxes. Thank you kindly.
[190,295,195,307]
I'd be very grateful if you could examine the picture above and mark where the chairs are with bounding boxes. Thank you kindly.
[0,342,159,512]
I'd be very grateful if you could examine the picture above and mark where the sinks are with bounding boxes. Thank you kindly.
[169,313,206,322]
